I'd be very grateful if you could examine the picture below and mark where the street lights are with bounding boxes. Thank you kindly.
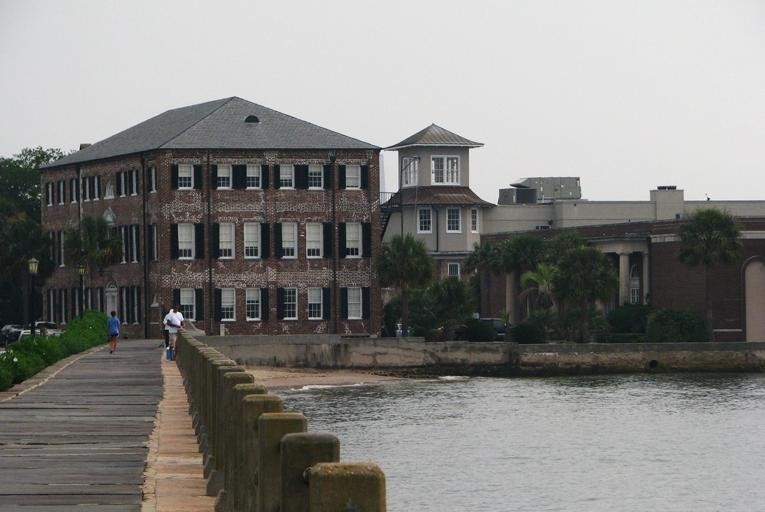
[27,257,39,339]
[77,262,85,321]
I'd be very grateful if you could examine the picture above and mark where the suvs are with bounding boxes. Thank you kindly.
[469,318,517,341]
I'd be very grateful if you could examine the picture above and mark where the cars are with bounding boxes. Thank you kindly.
[426,324,468,341]
[379,323,416,337]
[0,321,65,366]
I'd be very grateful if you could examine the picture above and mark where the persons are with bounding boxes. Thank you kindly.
[107,311,121,353]
[159,304,184,361]
